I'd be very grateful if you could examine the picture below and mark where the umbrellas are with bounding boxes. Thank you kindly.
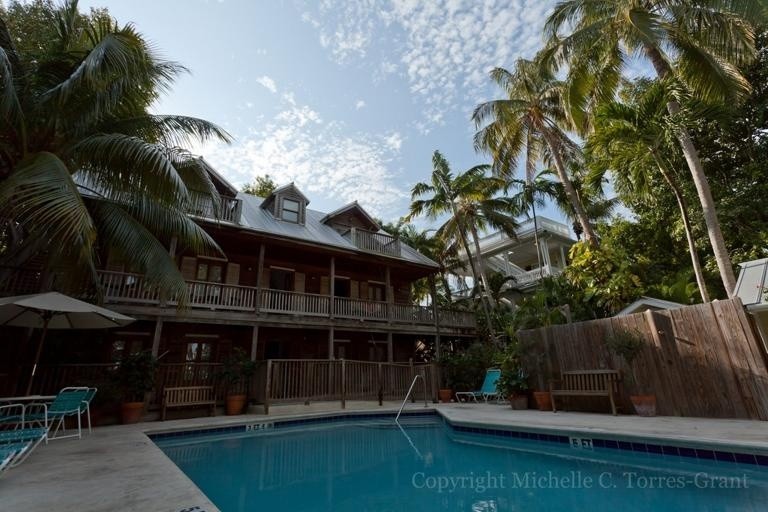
[0,287,138,395]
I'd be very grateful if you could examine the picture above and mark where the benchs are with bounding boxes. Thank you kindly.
[547,369,629,416]
[162,385,217,420]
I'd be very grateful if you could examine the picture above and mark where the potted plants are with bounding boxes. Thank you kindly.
[493,341,529,410]
[600,330,657,417]
[109,350,160,424]
[221,346,255,415]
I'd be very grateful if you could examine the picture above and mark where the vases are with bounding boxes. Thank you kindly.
[439,390,451,403]
[533,391,553,411]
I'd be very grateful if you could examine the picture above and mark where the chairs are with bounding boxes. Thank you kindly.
[1,386,97,478]
[455,368,524,405]
